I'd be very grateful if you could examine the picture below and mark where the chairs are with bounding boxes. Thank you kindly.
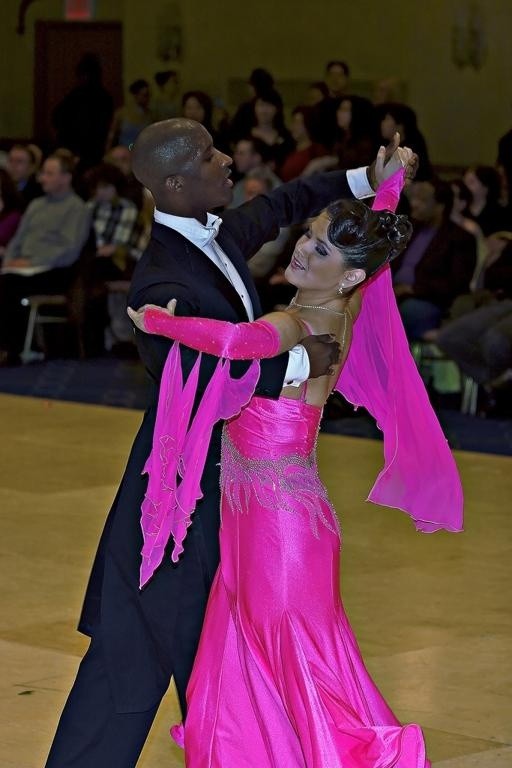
[0,170,511,415]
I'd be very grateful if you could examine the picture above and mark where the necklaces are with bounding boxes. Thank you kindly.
[285,289,353,343]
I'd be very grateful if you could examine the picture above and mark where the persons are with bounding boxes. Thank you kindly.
[1,56,511,418]
[40,116,423,768]
[126,143,466,768]
